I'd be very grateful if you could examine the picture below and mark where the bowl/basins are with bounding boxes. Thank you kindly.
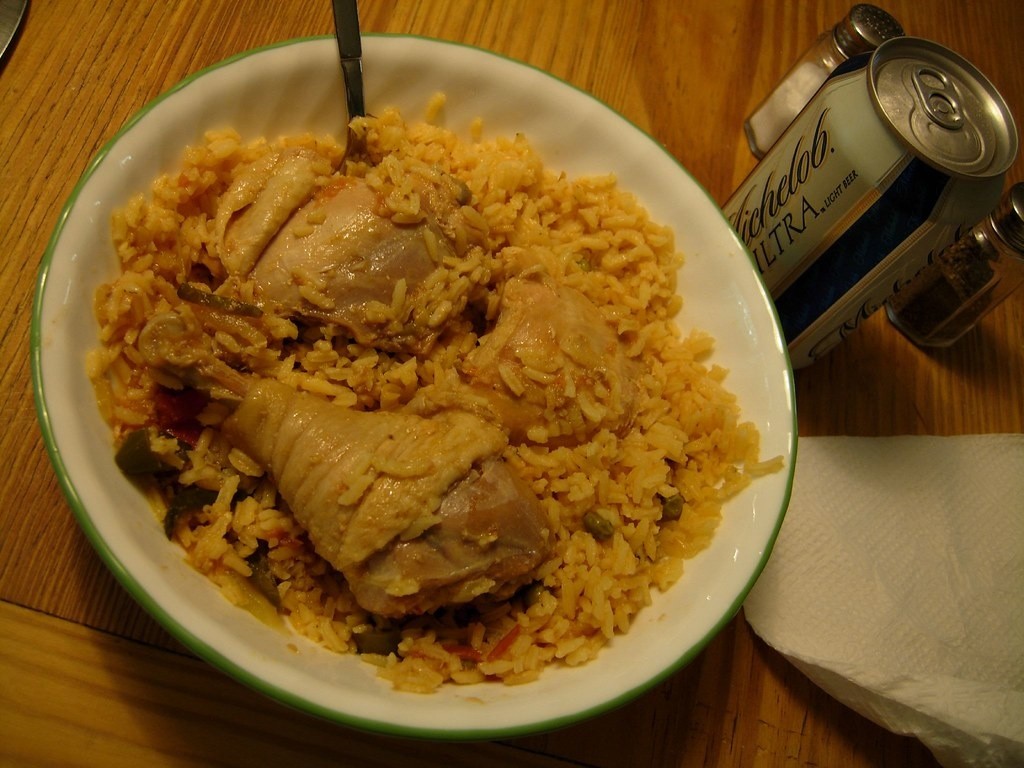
[32,31,798,737]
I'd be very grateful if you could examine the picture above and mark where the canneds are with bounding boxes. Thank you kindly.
[723,37,1019,365]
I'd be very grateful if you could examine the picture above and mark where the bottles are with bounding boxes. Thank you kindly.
[884,181,1024,347]
[743,4,907,159]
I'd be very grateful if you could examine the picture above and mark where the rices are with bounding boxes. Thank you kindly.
[90,95,783,690]
